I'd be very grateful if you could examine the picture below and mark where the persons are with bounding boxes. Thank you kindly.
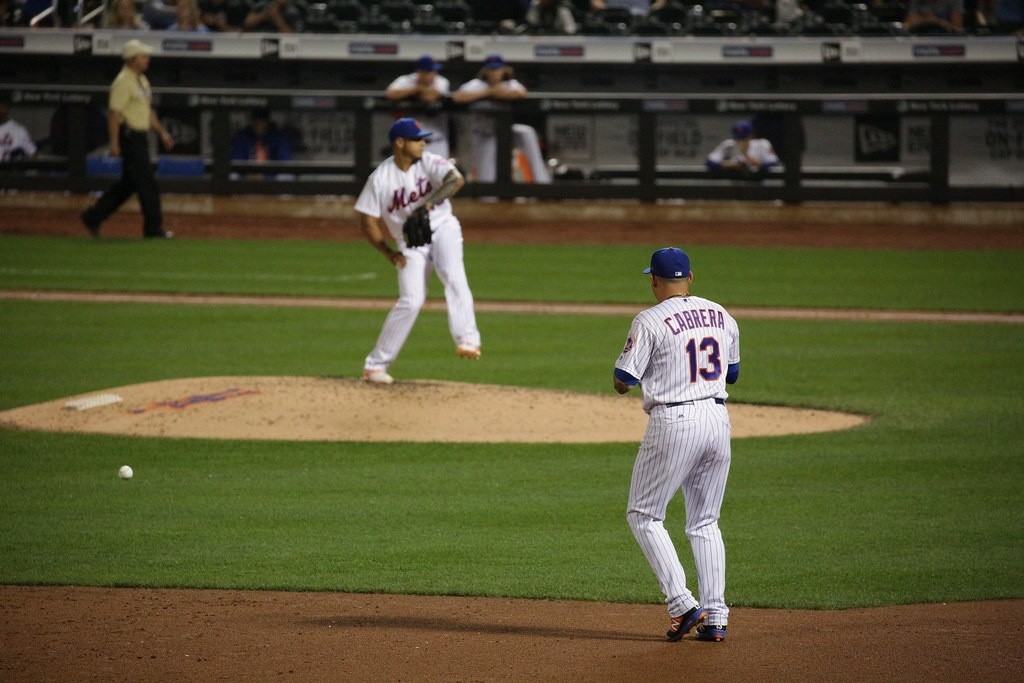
[78,39,177,239]
[1,0,1024,203]
[615,247,741,641]
[354,117,480,386]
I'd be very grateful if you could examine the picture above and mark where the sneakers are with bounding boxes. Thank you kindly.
[696,625,726,642]
[457,343,481,361]
[664,605,708,642]
[364,369,395,386]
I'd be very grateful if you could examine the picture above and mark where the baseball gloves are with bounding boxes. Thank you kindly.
[402,205,436,249]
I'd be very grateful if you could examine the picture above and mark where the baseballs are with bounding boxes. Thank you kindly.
[118,465,134,479]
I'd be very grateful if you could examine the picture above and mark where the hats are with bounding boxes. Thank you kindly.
[643,247,690,278]
[389,119,432,144]
[123,39,154,59]
[484,57,504,69]
[732,120,754,139]
[418,55,443,72]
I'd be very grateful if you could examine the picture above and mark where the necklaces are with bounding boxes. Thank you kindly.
[667,293,690,299]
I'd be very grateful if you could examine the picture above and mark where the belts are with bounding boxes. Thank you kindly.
[666,398,725,407]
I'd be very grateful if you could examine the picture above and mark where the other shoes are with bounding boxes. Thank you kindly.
[149,231,175,240]
[484,196,500,205]
[79,212,100,238]
[536,173,552,185]
